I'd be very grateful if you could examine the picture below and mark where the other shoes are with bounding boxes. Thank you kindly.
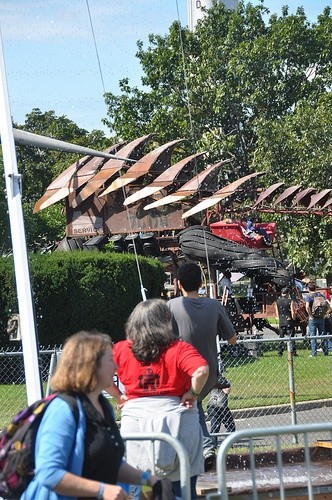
[310,354,317,358]
[205,452,216,472]
[323,352,328,356]
[278,351,282,355]
[293,353,298,356]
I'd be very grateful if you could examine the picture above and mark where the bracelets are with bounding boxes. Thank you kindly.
[188,387,199,398]
[98,481,104,499]
[141,472,148,482]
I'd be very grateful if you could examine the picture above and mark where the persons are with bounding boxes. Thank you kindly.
[18,329,165,500]
[276,287,300,357]
[305,283,332,356]
[103,298,210,500]
[239,217,272,248]
[205,387,236,449]
[166,262,237,474]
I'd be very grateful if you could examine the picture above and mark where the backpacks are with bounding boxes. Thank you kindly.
[312,297,328,317]
[0,392,71,499]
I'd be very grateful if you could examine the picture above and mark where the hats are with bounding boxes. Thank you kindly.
[281,288,290,294]
[302,278,310,283]
